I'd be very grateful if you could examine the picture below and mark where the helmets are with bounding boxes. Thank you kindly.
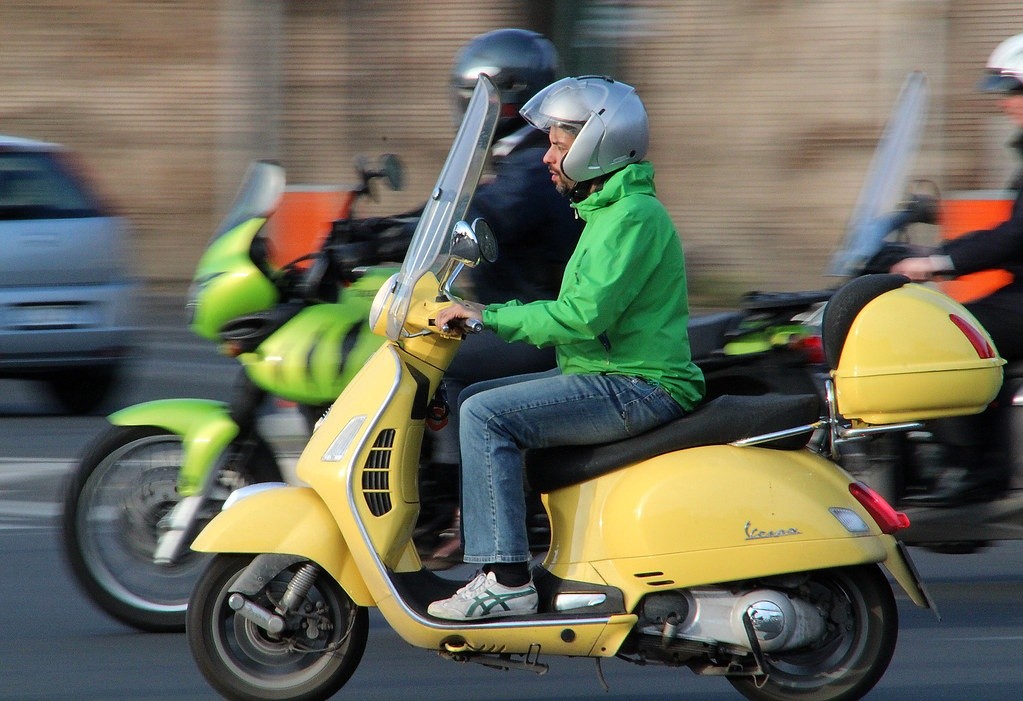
[452,28,561,103]
[985,33,1023,82]
[539,75,649,182]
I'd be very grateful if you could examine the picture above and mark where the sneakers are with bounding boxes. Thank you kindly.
[427,569,539,621]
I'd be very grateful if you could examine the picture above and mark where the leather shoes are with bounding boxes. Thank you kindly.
[898,471,1007,506]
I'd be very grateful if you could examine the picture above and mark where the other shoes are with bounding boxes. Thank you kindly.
[432,506,460,558]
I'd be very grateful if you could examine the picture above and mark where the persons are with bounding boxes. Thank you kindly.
[322,29,587,548]
[427,74,705,618]
[891,33,1023,509]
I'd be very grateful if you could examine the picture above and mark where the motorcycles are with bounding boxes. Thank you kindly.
[55,155,834,632]
[181,263,1007,701]
[690,197,1023,553]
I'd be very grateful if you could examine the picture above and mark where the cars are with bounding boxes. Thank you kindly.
[0,125,140,418]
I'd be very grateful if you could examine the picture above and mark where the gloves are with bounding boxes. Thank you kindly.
[322,241,379,282]
